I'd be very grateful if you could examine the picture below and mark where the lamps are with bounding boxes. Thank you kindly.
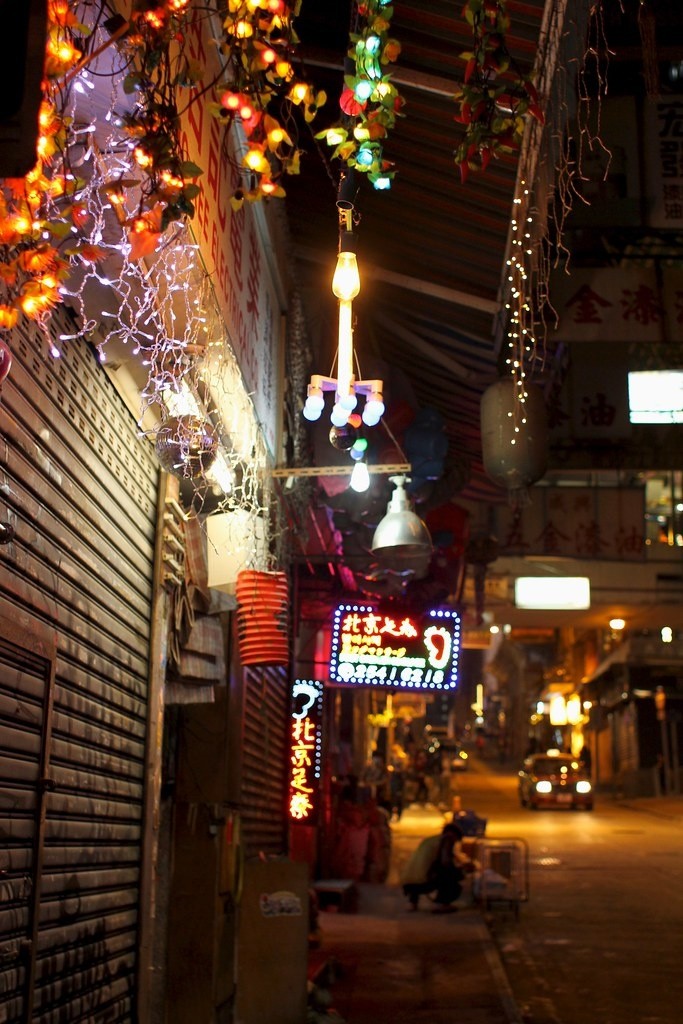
[164,381,234,497]
[302,167,385,492]
[371,476,434,559]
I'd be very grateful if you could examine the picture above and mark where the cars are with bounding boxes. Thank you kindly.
[437,740,468,772]
[516,752,594,810]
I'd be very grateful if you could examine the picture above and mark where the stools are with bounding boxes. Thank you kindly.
[313,880,355,914]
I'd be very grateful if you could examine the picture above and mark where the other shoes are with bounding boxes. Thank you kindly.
[405,902,417,912]
[430,903,458,914]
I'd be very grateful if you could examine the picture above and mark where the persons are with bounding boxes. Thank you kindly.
[414,752,429,801]
[359,750,390,802]
[580,747,592,776]
[387,759,405,818]
[401,823,480,913]
[525,737,540,757]
